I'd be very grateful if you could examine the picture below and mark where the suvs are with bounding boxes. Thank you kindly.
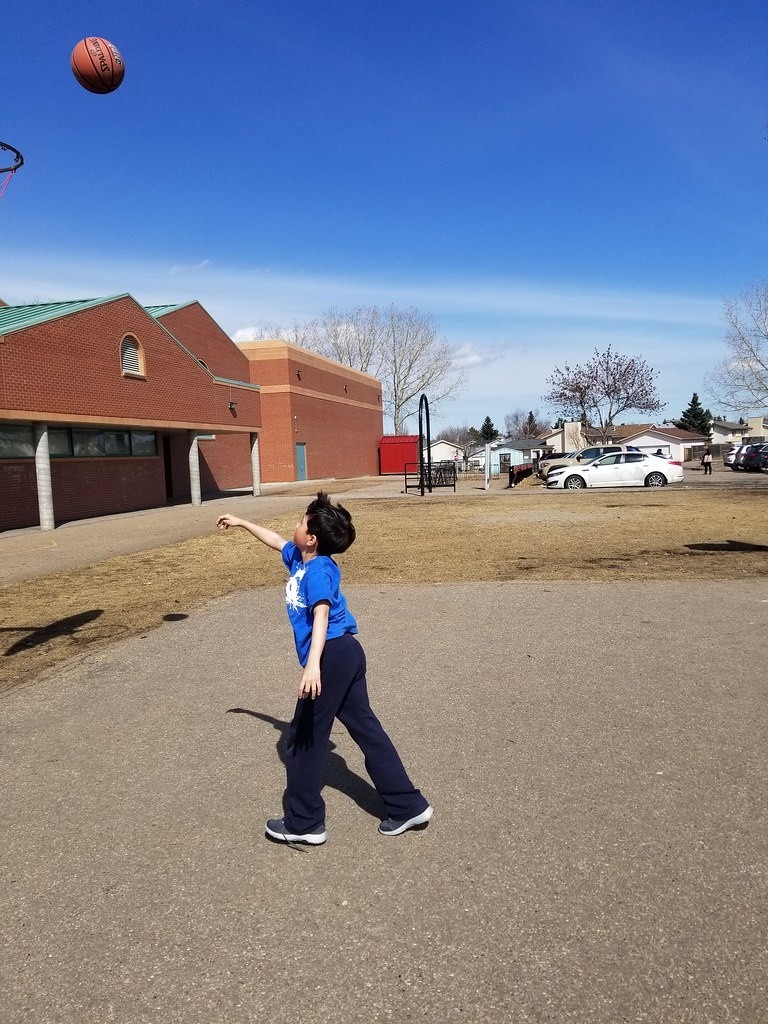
[723,441,768,472]
[536,445,673,480]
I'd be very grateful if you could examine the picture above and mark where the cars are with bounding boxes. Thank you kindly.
[547,452,685,490]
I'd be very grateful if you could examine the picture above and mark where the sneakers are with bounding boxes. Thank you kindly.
[265,818,326,854]
[379,806,434,836]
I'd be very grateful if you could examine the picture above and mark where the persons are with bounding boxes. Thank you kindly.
[217,492,434,852]
[701,449,712,475]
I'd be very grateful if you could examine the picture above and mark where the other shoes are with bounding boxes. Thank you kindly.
[703,473,707,475]
[709,473,711,475]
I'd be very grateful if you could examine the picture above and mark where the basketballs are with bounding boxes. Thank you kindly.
[71,38,126,97]
